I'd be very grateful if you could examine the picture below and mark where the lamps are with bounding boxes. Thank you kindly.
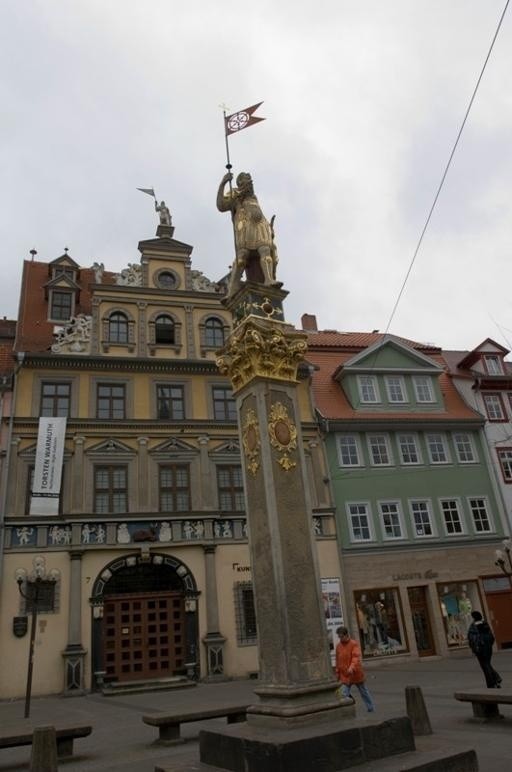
[182,587,202,615]
[88,595,105,621]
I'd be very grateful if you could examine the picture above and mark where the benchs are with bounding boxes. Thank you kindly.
[450,685,511,721]
[1,702,254,761]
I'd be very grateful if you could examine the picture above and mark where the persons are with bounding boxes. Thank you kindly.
[155,200,172,227]
[467,611,502,688]
[334,626,376,711]
[357,593,391,648]
[440,588,473,639]
[217,172,284,304]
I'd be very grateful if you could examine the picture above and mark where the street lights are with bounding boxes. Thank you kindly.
[15,556,61,719]
[495,539,512,589]
